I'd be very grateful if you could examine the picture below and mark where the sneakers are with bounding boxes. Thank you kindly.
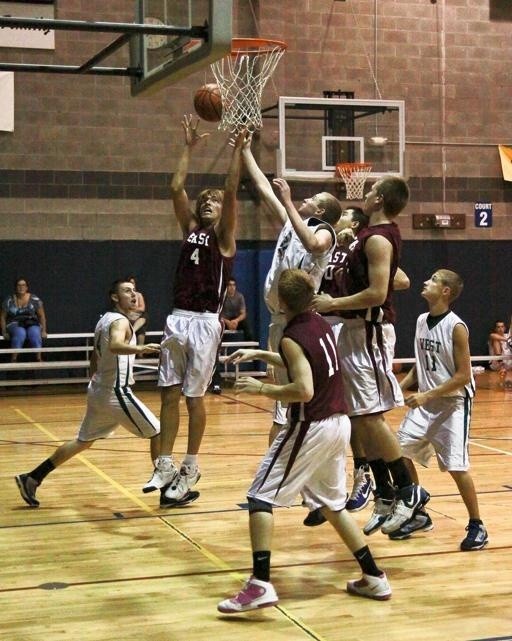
[218,576,279,614]
[15,474,39,507]
[303,466,488,551]
[142,457,201,509]
[347,571,391,600]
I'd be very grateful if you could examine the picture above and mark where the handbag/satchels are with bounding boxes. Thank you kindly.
[19,316,36,328]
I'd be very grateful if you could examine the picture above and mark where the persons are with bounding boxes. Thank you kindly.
[13,278,201,510]
[139,113,489,553]
[484,320,512,380]
[123,277,148,360]
[0,277,49,364]
[213,266,395,614]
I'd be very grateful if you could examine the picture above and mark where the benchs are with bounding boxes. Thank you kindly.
[0,329,267,397]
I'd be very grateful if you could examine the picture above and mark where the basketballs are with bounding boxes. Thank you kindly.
[194,83,230,121]
